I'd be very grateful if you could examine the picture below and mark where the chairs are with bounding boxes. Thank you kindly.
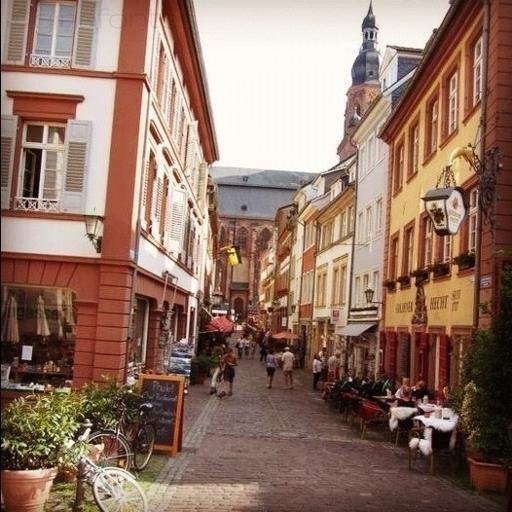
[339,390,455,475]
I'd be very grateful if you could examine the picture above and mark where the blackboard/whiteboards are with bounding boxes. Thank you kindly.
[138,375,184,451]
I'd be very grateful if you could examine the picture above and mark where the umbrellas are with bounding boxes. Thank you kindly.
[271,330,299,345]
[33,295,51,336]
[0,295,20,343]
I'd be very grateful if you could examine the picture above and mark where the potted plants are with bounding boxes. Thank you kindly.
[0,373,143,512]
[457,326,512,493]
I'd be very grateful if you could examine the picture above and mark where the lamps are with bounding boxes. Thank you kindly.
[365,288,384,306]
[82,213,105,253]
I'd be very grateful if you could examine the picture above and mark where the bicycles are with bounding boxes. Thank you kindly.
[65,392,157,512]
[210,358,223,394]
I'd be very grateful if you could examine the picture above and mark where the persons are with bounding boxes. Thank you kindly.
[236,335,305,368]
[218,348,238,398]
[312,348,341,391]
[282,346,295,390]
[208,345,224,394]
[394,377,431,429]
[265,350,278,389]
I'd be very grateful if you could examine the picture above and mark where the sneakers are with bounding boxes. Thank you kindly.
[209,387,217,395]
[218,391,225,398]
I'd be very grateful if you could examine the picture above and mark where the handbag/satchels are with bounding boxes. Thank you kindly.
[223,364,235,378]
